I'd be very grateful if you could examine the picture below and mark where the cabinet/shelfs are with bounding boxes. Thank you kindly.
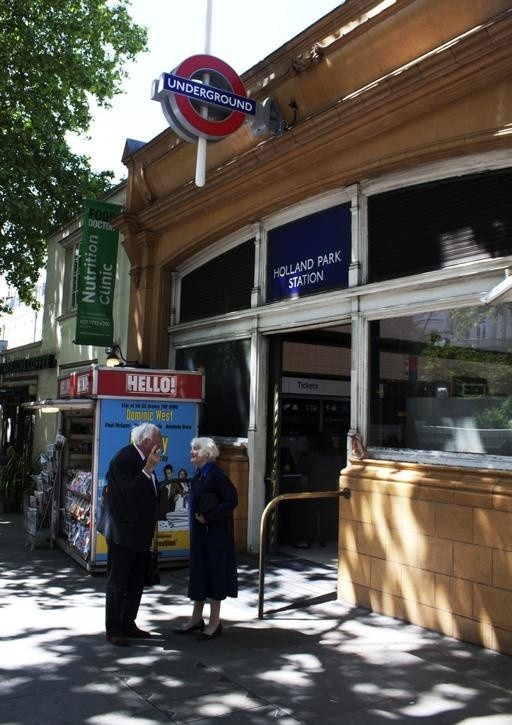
[65,418,94,460]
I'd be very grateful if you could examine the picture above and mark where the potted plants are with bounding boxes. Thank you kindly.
[0,453,31,513]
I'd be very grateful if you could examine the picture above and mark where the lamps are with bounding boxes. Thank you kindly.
[105,346,140,368]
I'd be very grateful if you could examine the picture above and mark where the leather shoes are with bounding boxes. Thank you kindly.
[106,626,150,646]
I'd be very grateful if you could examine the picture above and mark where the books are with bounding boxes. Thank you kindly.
[22,434,92,560]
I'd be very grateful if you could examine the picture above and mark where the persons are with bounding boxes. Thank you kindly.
[171,437,239,642]
[100,470,109,515]
[158,464,183,513]
[174,468,190,513]
[95,422,161,647]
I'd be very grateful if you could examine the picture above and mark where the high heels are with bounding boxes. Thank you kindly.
[173,619,222,641]
